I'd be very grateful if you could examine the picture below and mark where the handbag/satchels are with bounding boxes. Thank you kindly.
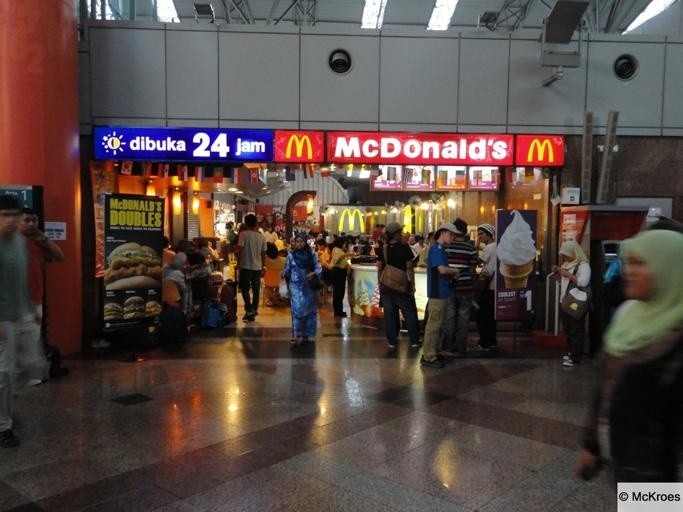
[379,265,408,294]
[471,276,490,293]
[309,266,322,290]
[561,280,593,322]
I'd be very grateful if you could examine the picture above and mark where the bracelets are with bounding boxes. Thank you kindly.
[40,236,48,244]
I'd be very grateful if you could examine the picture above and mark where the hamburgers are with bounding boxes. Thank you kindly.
[104,242,162,290]
[104,296,162,321]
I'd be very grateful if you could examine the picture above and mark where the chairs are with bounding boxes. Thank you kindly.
[193,231,417,307]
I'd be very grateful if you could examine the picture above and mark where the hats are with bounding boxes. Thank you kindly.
[437,223,462,235]
[386,222,406,235]
[478,223,495,240]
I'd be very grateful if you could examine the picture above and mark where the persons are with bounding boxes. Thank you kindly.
[17,207,67,387]
[574,217,682,493]
[161,212,495,367]
[550,240,592,368]
[0,194,50,449]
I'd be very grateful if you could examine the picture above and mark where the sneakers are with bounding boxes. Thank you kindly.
[422,358,445,368]
[289,337,308,348]
[334,311,347,317]
[562,359,576,366]
[411,337,423,347]
[0,429,20,448]
[466,344,490,351]
[243,307,257,320]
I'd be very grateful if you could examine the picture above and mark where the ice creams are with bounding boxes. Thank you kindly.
[370,282,382,317]
[360,281,369,316]
[496,209,536,289]
